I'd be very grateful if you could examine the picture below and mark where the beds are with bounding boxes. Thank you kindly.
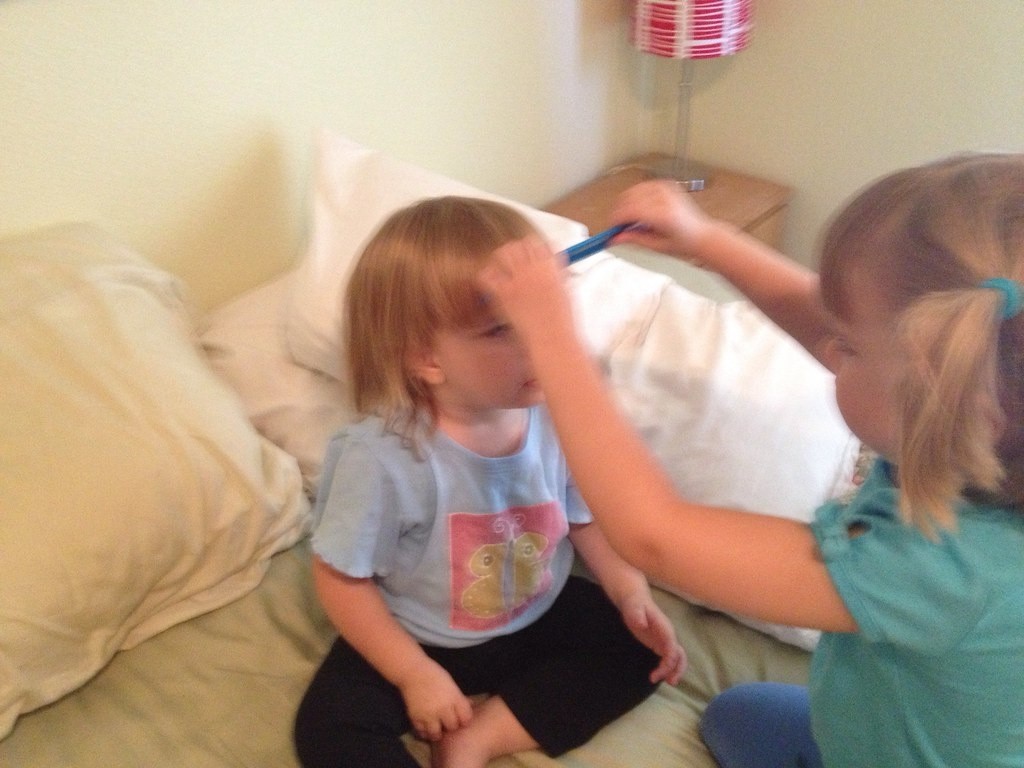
[0,242,821,767]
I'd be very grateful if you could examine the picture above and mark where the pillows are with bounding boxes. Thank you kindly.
[0,128,863,749]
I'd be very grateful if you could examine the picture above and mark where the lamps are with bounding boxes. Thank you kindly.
[633,0,757,194]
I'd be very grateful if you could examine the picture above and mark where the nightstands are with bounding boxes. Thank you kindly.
[538,150,797,279]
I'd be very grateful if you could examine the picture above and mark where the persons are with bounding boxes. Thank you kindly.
[294,195,688,768]
[480,153,1024,768]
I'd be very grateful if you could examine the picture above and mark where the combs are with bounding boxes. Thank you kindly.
[551,179,704,269]
